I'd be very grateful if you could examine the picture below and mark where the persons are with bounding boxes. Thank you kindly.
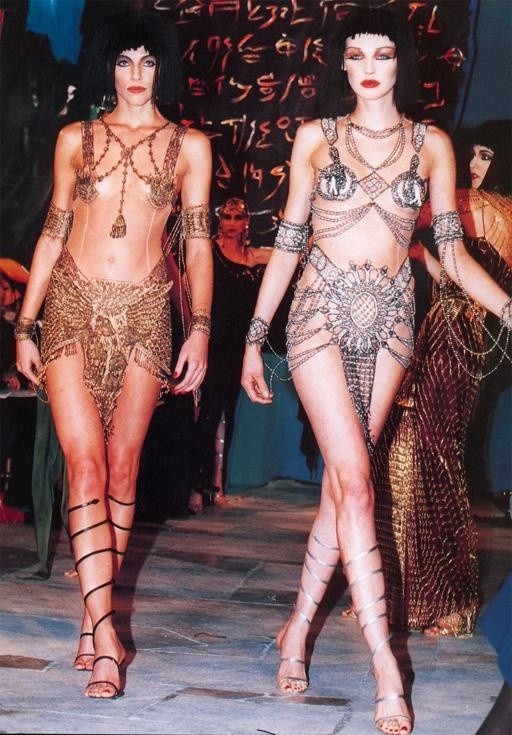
[0,255,46,525]
[12,18,216,705]
[239,15,511,734]
[176,195,278,522]
[338,116,510,643]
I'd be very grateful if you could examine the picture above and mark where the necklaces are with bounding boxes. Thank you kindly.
[342,106,408,204]
[95,114,180,241]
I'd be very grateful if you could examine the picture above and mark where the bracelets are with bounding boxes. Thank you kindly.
[13,315,41,346]
[243,317,272,357]
[186,305,212,339]
[497,297,510,332]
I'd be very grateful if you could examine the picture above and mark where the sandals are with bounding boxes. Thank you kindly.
[374,668,412,735]
[73,626,126,698]
[276,631,309,695]
